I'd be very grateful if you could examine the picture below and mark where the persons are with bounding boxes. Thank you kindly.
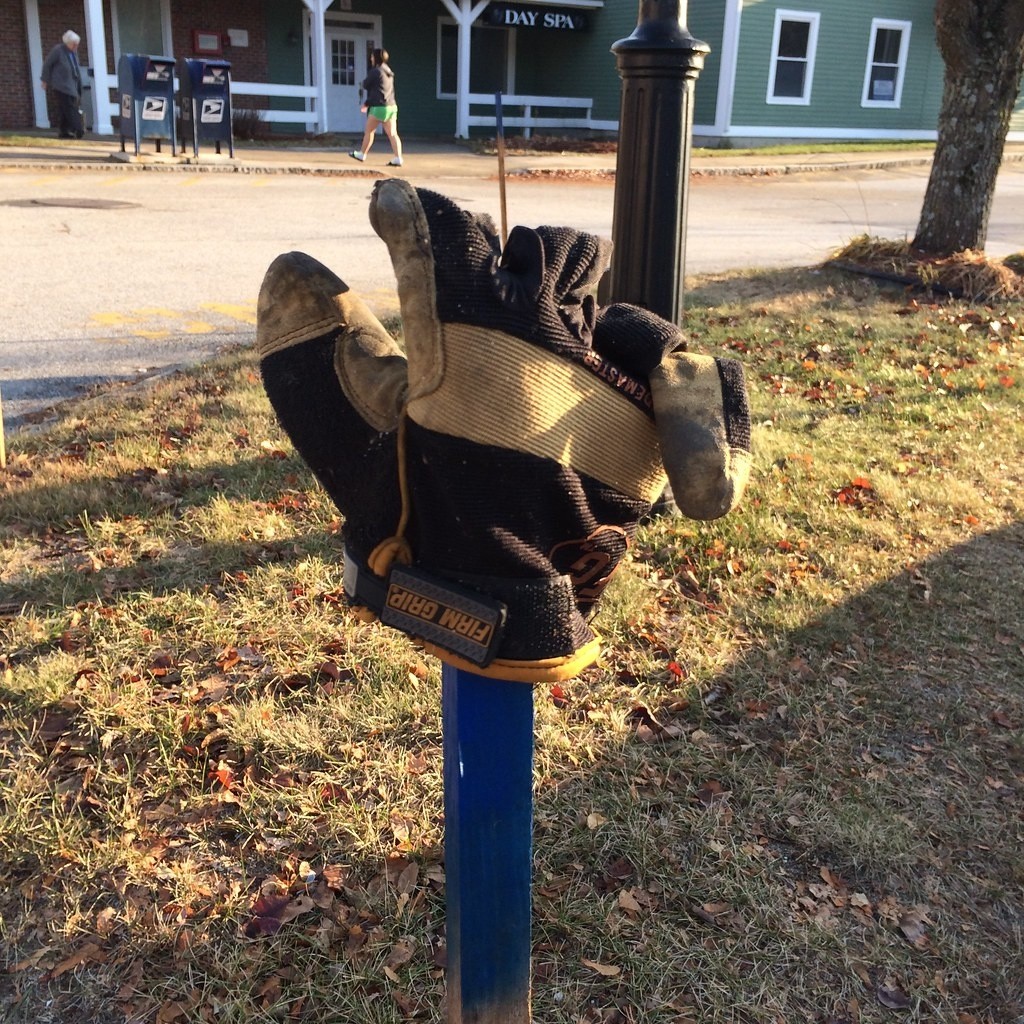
[41,30,85,139]
[349,49,402,166]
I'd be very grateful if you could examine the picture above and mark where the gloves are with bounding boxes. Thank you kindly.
[259,178,748,679]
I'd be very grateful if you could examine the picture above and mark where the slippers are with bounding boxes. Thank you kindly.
[349,150,364,162]
[385,161,402,166]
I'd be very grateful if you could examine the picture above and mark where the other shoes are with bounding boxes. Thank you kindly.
[76,131,84,138]
[59,133,74,139]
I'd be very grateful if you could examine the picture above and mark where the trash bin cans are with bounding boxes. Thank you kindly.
[116,52,178,159]
[176,55,234,159]
